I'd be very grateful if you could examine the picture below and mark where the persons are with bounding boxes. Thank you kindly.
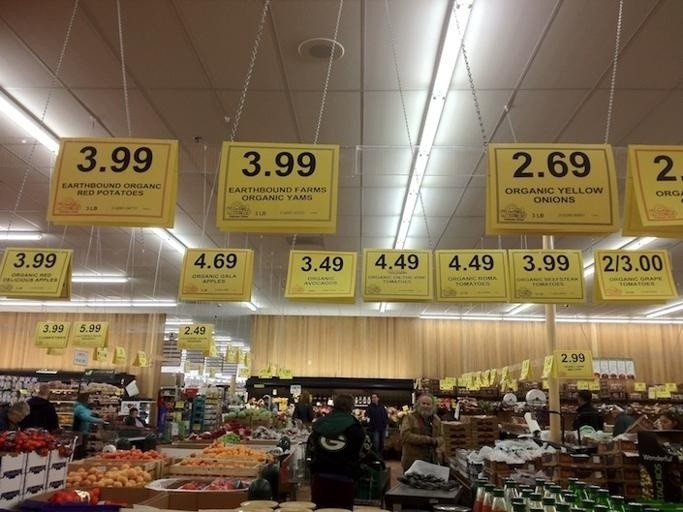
[125,407,146,429]
[72,392,105,460]
[306,393,366,512]
[573,390,604,432]
[20,384,59,432]
[660,410,682,430]
[0,401,30,432]
[399,393,446,476]
[611,403,635,439]
[364,391,388,456]
[292,393,313,425]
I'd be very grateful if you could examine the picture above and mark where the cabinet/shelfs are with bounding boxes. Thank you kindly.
[0,437,77,512]
[157,383,231,443]
[385,478,461,512]
[0,367,158,452]
[245,375,418,444]
[561,379,683,499]
[419,378,552,459]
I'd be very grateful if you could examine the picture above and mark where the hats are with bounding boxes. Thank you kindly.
[615,401,626,413]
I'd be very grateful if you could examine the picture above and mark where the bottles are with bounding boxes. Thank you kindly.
[519,484,533,495]
[506,481,518,512]
[491,490,507,512]
[511,493,543,512]
[473,482,483,512]
[355,394,371,404]
[482,486,492,512]
[537,477,659,511]
